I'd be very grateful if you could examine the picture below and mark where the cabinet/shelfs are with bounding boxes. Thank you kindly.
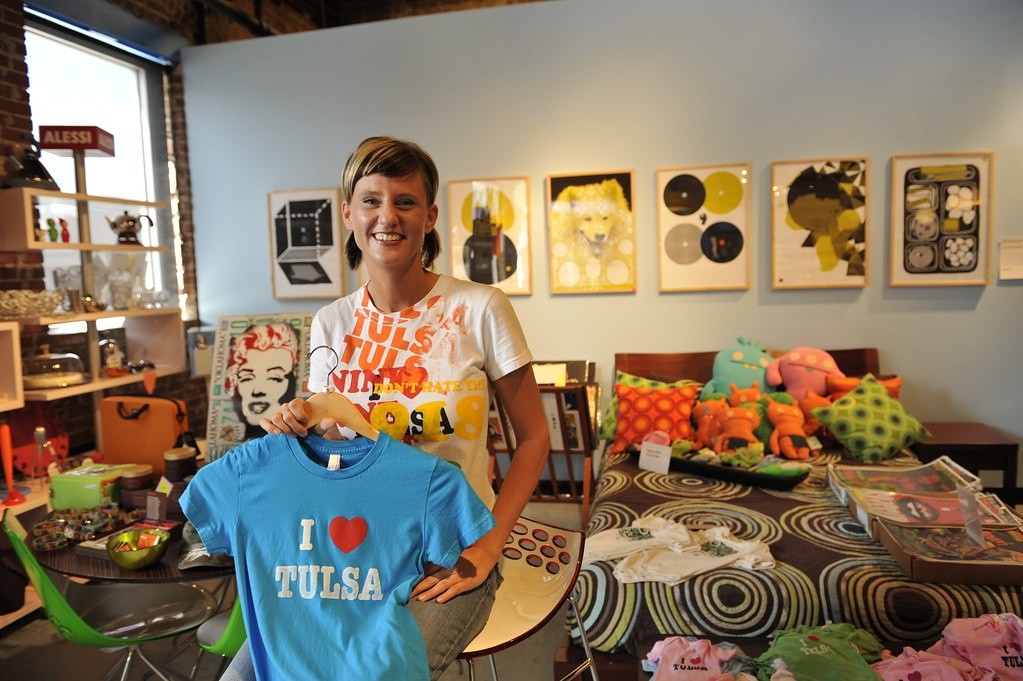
[0,187,187,450]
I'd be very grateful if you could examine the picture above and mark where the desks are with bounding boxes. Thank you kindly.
[22,516,235,681]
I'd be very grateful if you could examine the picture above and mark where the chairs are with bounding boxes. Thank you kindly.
[2,508,219,681]
[189,594,250,681]
[456,518,598,681]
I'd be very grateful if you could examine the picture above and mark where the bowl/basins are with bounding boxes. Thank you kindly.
[105,526,171,571]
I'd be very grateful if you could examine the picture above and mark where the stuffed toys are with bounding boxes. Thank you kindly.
[632,337,845,471]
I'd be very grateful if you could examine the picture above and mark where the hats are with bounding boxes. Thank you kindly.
[177,519,234,569]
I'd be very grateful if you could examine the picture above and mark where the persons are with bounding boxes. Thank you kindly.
[217,136,552,681]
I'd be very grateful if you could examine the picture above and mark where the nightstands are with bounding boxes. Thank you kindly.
[912,422,1019,504]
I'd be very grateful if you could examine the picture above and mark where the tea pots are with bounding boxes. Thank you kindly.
[105,210,153,234]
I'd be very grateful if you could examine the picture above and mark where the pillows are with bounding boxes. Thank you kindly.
[812,372,933,461]
[825,374,904,402]
[600,369,704,442]
[612,384,699,454]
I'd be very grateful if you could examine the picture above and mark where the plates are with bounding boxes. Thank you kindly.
[1,406,68,478]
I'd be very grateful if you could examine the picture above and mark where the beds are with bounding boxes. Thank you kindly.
[552,345,1023,681]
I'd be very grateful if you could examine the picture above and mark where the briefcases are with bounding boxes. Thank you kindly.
[100,396,187,475]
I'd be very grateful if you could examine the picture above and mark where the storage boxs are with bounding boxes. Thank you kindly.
[50,464,137,513]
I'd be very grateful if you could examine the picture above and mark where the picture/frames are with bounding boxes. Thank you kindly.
[546,169,637,294]
[772,156,871,289]
[268,187,346,300]
[657,163,752,294]
[889,152,996,288]
[450,177,533,296]
[357,248,436,290]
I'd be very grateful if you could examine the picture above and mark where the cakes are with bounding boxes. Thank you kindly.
[23,372,84,389]
